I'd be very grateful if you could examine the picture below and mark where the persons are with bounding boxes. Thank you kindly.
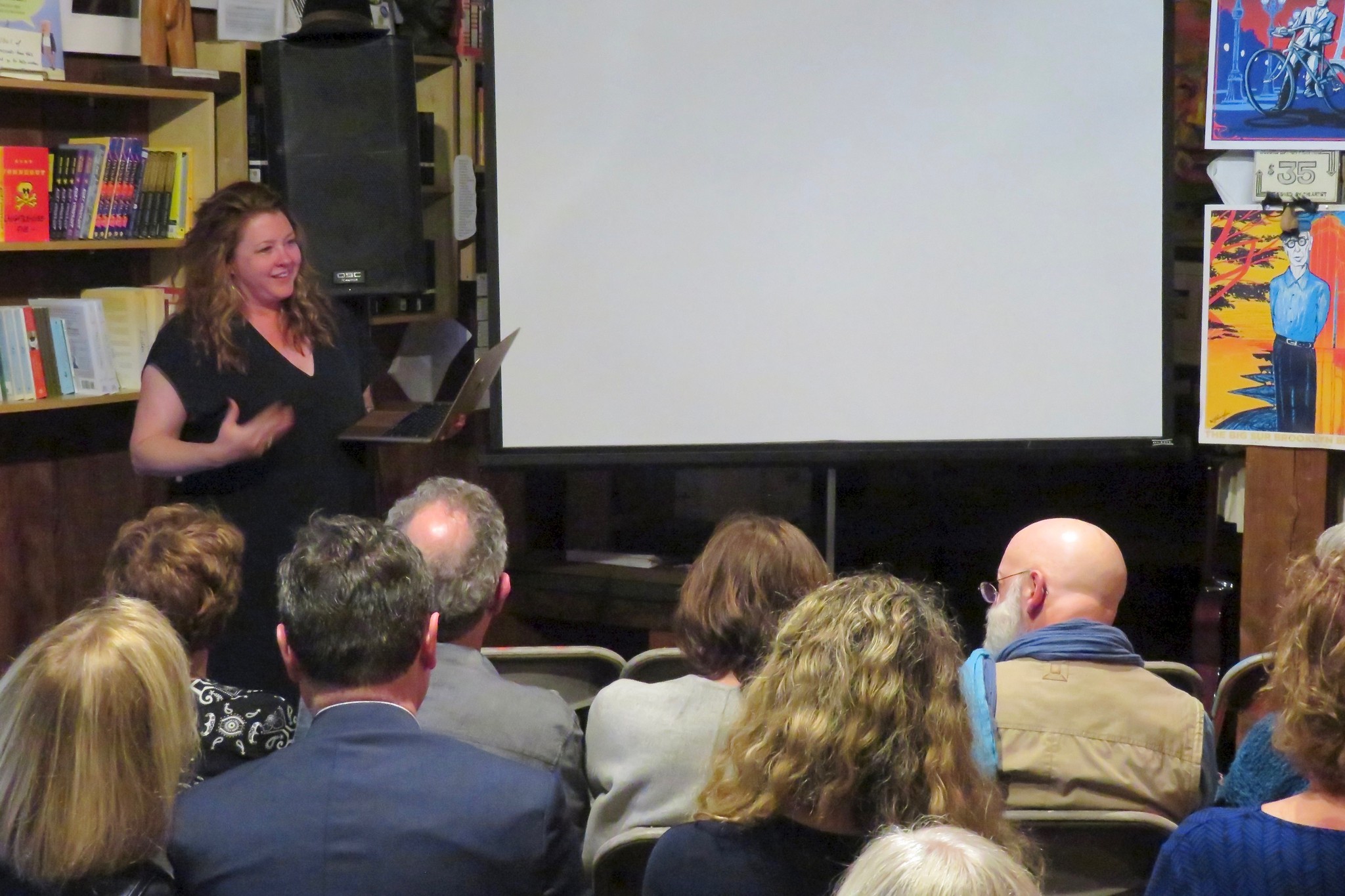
[128,181,465,714]
[0,475,1344,896]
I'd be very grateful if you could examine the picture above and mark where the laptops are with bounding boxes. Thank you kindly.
[338,328,520,444]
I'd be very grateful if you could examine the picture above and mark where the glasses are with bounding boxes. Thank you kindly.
[980,568,1050,605]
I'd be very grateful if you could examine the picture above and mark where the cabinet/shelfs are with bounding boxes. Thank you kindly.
[0,33,484,416]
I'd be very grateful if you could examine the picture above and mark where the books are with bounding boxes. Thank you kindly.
[0,138,196,405]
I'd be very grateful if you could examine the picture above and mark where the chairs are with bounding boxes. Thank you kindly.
[323,637,1345,896]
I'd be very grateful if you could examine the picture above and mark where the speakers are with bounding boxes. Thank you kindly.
[261,32,428,299]
